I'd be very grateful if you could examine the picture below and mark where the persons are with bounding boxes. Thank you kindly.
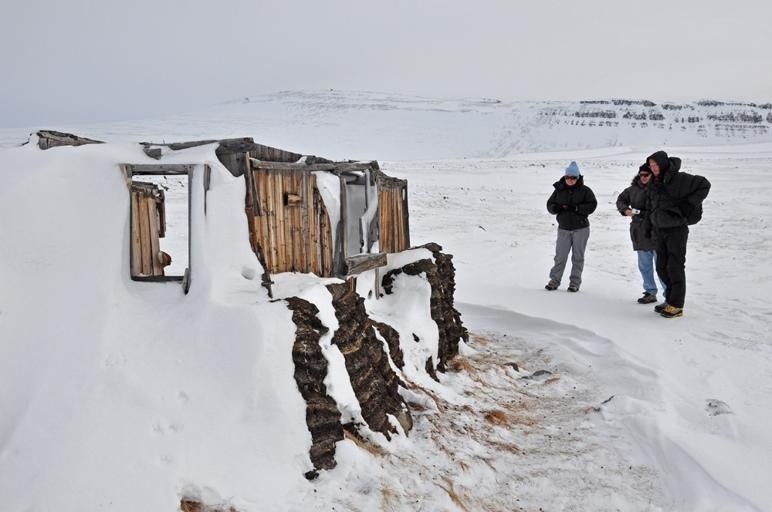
[616,162,667,304]
[645,150,711,317]
[544,161,597,292]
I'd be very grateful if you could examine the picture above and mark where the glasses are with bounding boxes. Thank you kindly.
[638,171,652,177]
[564,175,578,180]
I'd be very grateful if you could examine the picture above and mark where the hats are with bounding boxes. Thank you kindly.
[564,161,581,177]
[639,162,652,174]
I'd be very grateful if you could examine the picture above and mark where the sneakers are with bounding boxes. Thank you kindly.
[567,284,579,292]
[638,291,658,304]
[654,301,684,319]
[545,283,558,291]
